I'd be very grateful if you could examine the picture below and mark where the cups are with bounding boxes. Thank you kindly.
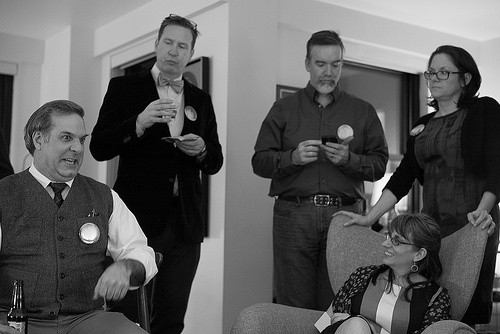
[161,99,178,122]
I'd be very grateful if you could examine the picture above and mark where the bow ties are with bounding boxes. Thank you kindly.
[157,76,185,95]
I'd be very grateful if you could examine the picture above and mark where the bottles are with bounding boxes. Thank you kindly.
[7,277,29,333]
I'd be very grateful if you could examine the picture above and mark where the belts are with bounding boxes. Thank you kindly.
[277,194,363,208]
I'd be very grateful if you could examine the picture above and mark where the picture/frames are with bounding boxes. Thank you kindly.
[277,83,303,101]
[182,56,209,93]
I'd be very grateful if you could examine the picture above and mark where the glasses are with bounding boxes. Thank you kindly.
[384,232,421,247]
[422,70,464,81]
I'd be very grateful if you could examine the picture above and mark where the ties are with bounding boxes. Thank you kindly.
[48,182,68,208]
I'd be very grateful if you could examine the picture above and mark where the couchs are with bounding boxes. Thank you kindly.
[229,214,491,334]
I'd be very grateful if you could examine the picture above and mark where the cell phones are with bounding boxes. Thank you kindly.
[321,135,338,145]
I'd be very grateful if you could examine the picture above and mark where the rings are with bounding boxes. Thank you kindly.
[330,153,334,159]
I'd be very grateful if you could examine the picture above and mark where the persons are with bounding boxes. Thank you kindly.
[1,99,159,334]
[84,16,224,334]
[329,43,500,326]
[321,213,453,334]
[250,30,392,312]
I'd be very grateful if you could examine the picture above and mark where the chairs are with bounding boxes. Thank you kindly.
[105,249,163,334]
